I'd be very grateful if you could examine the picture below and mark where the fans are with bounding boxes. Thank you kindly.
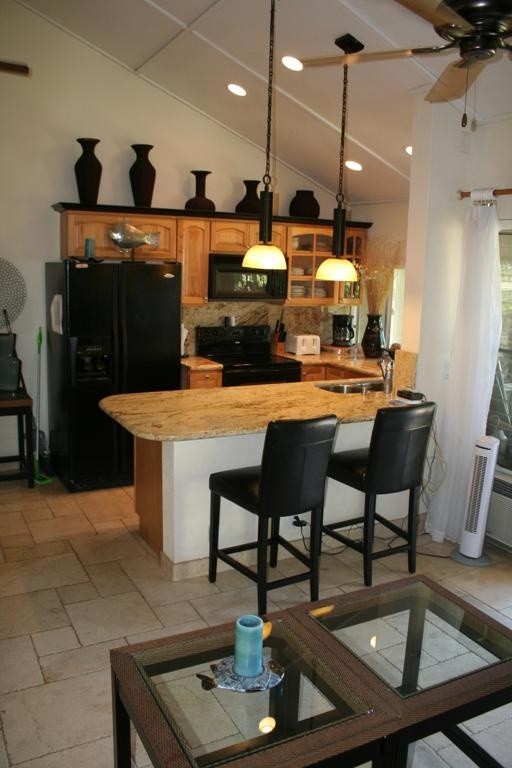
[299,0,512,105]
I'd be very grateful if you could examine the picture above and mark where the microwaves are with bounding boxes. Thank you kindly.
[208,253,289,302]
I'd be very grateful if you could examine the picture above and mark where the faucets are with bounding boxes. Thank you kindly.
[376,352,393,394]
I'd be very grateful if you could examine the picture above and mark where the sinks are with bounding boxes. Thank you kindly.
[353,378,386,392]
[315,381,369,396]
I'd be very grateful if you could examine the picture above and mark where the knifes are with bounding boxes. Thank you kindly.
[273,318,289,342]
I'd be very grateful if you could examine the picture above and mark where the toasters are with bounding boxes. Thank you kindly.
[284,332,321,355]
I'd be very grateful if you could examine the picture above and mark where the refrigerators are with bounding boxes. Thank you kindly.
[44,256,185,497]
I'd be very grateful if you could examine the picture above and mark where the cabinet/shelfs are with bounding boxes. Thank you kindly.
[301,363,384,382]
[51,202,177,261]
[177,214,211,308]
[211,212,286,255]
[286,217,373,306]
[181,367,223,390]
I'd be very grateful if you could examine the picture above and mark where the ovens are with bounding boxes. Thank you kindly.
[220,368,302,387]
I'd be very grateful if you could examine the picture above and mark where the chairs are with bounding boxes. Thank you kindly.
[204,415,340,614]
[321,402,436,587]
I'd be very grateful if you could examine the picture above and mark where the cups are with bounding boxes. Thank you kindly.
[234,613,264,677]
[225,316,235,326]
[83,237,95,258]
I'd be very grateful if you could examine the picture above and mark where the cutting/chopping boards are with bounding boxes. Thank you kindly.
[392,348,416,397]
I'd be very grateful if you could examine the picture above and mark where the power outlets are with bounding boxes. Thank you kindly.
[288,515,304,529]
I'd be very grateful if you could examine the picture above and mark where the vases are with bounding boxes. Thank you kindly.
[361,313,386,357]
[183,169,217,211]
[286,189,320,217]
[235,179,264,213]
[128,143,158,206]
[72,135,102,204]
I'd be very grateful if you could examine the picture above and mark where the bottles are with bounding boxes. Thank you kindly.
[74,135,321,220]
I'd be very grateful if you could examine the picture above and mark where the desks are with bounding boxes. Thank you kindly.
[0,388,37,486]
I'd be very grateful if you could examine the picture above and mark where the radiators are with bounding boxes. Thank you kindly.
[485,471,511,548]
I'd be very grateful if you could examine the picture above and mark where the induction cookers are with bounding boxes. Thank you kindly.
[195,352,301,372]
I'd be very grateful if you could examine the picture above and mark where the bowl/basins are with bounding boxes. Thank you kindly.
[290,236,332,299]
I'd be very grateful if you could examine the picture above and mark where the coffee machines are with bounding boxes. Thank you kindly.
[331,313,356,348]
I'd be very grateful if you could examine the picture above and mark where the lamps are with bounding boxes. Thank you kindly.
[243,0,291,273]
[316,56,358,281]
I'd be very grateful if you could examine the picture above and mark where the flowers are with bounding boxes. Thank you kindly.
[350,258,394,314]
